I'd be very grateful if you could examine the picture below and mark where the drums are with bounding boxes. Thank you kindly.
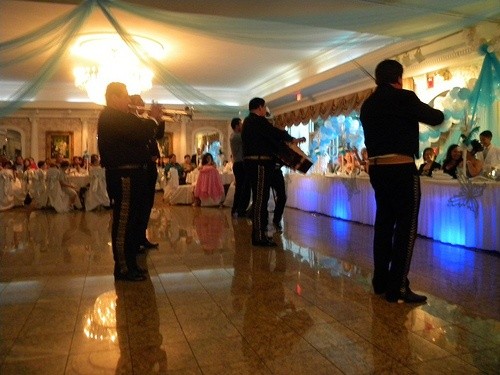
[275,130,313,174]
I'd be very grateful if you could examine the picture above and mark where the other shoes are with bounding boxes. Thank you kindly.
[273,220,283,231]
[137,236,159,250]
[231,209,253,218]
[374,281,429,305]
[218,202,224,209]
[192,200,198,206]
[114,266,151,283]
[251,236,279,248]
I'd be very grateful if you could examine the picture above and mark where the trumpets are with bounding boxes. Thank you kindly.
[129,104,193,124]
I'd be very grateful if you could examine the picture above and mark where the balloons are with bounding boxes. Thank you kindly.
[309,38,500,162]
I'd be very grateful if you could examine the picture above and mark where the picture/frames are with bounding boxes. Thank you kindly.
[156,132,174,158]
[44,130,75,165]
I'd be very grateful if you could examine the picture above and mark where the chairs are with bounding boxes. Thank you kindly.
[0,167,235,211]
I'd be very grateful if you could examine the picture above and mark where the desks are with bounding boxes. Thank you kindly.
[283,169,500,252]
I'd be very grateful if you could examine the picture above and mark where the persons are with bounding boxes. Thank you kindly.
[1,210,233,260]
[240,97,299,248]
[97,83,149,282]
[366,298,422,375]
[111,279,169,374]
[360,60,445,304]
[126,95,165,251]
[229,117,255,219]
[230,221,313,374]
[0,129,499,215]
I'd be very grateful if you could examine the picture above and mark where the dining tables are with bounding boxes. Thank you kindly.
[65,168,89,192]
[186,166,234,186]
